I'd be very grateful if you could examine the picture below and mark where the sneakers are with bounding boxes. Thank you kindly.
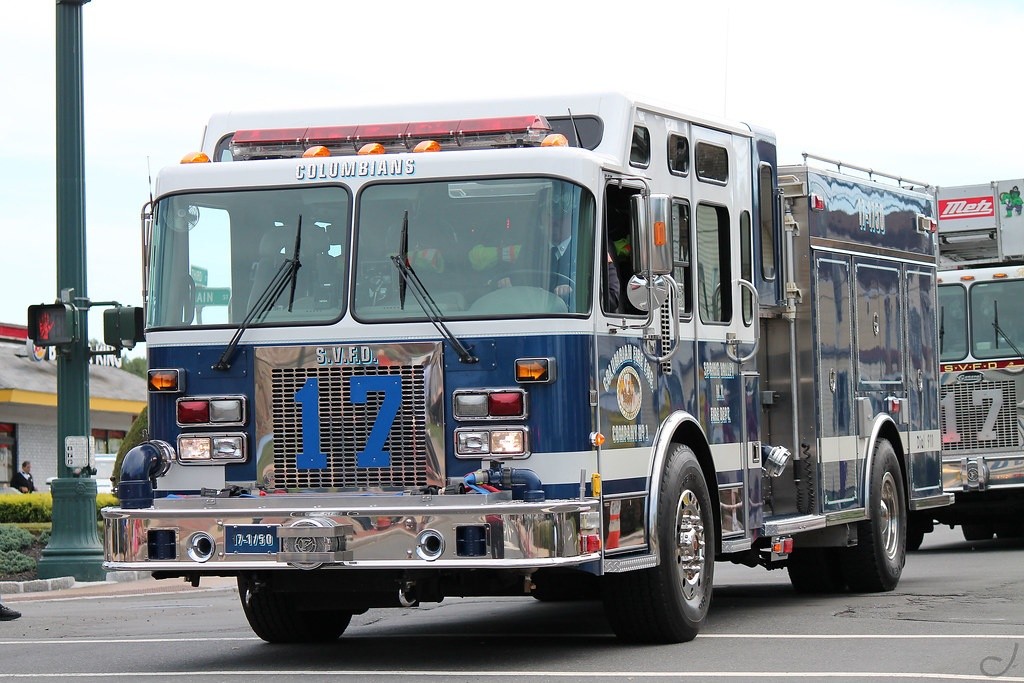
[0,605,21,622]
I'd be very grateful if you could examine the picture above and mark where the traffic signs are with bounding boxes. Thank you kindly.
[189,265,231,306]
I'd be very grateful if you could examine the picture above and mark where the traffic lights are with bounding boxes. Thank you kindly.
[24,300,74,346]
[103,307,146,348]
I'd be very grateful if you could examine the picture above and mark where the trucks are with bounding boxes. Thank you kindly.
[940,178,1018,543]
[100,68,957,646]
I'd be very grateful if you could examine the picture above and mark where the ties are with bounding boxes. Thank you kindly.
[551,246,558,268]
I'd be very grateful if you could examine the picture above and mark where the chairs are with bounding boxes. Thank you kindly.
[247,224,337,318]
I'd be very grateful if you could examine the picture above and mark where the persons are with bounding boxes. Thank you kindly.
[10,461,35,493]
[0,603,22,621]
[497,198,620,312]
